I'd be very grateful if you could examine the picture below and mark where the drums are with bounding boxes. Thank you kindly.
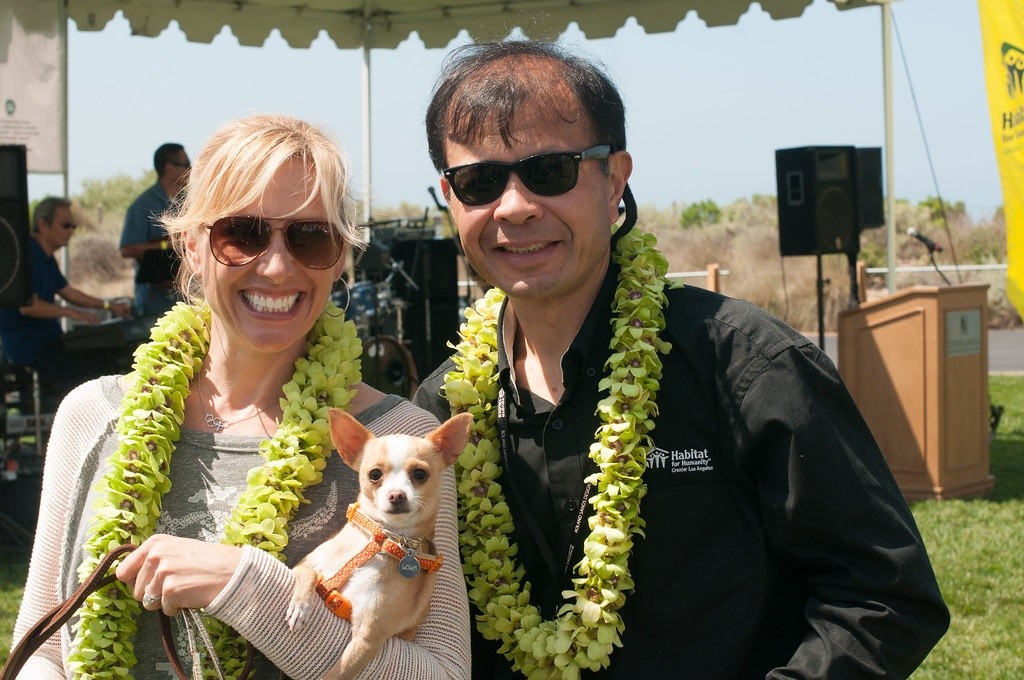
[362,335,418,400]
[330,280,377,328]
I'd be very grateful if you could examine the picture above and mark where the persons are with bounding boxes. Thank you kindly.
[0,117,472,680]
[414,42,952,680]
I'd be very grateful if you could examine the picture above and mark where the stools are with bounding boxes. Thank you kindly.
[0,362,54,456]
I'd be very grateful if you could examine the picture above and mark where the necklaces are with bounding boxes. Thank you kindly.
[196,371,285,432]
[437,226,673,679]
[71,303,362,679]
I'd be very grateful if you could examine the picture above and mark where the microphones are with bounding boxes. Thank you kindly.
[428,187,442,211]
[908,228,943,253]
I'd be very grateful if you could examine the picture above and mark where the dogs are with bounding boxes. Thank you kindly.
[284,408,474,680]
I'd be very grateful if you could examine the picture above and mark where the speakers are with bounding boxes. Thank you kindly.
[775,145,883,256]
[0,142,36,308]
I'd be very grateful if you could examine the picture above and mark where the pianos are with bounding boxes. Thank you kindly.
[60,311,168,351]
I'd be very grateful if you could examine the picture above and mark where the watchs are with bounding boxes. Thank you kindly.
[103,300,110,309]
[161,241,168,249]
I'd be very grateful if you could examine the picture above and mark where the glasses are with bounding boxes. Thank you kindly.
[205,218,345,270]
[443,145,614,206]
[168,160,191,169]
[61,222,77,229]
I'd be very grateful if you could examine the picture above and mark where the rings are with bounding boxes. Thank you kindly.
[140,589,160,606]
